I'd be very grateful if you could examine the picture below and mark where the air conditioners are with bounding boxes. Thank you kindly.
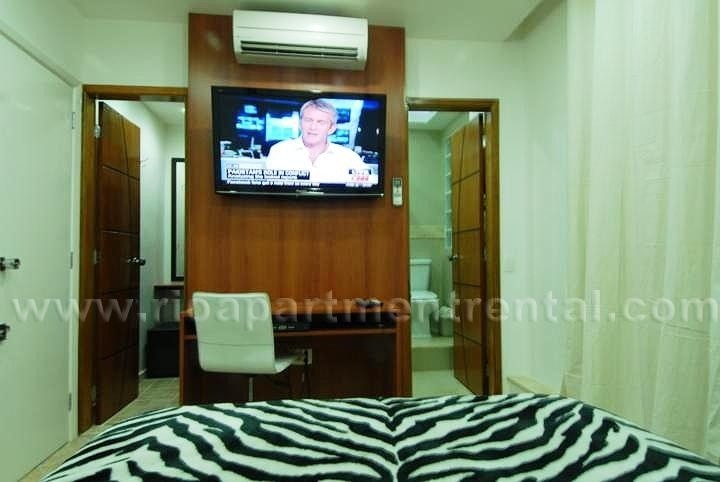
[232,9,369,72]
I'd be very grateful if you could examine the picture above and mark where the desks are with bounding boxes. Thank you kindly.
[176,297,411,400]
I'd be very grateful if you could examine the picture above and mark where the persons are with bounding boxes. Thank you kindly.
[265,99,368,186]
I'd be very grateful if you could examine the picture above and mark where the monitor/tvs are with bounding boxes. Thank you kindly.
[211,86,386,197]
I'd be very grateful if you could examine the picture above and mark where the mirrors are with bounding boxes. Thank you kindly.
[170,157,186,281]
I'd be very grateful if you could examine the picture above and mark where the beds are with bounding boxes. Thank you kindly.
[33,391,720,482]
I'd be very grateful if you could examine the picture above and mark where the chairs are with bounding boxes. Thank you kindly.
[192,291,299,402]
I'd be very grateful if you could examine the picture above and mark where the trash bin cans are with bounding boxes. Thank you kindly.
[441,307,454,336]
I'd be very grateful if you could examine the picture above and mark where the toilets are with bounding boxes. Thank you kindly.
[409,258,439,339]
